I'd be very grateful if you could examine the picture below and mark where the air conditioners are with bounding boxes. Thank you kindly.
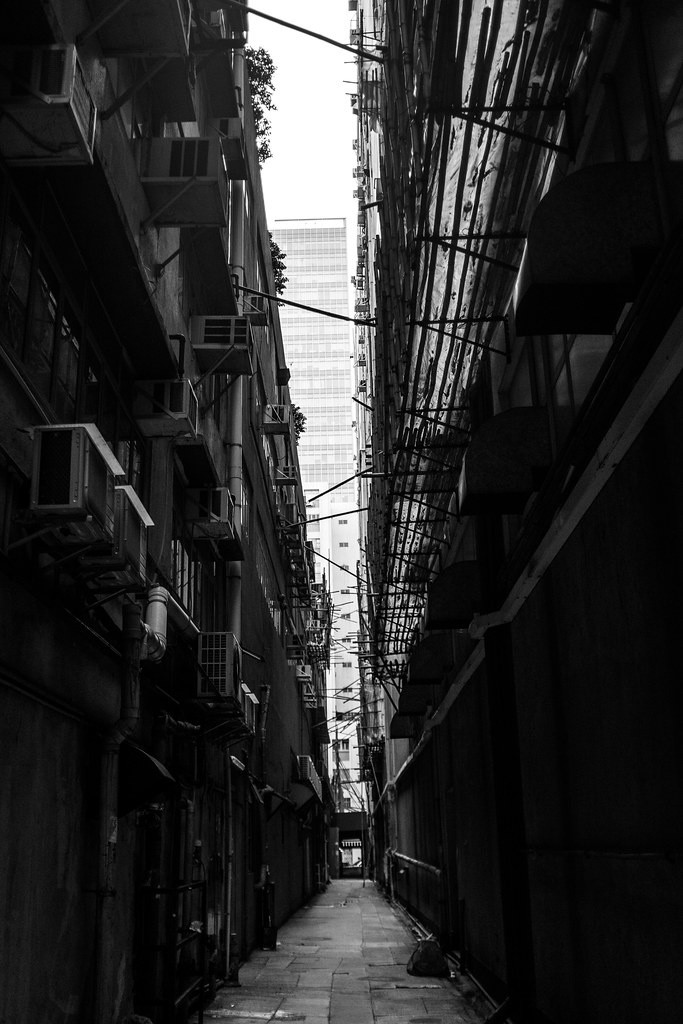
[243,295,270,326]
[296,665,312,680]
[206,117,245,162]
[210,12,225,37]
[210,488,235,539]
[148,59,200,124]
[299,756,317,780]
[192,315,253,373]
[144,139,230,229]
[30,424,115,540]
[79,488,147,587]
[287,505,299,529]
[263,404,292,434]
[50,0,192,58]
[276,466,299,484]
[213,687,256,735]
[196,632,242,706]
[0,46,96,165]
[135,378,198,436]
[307,619,321,632]
